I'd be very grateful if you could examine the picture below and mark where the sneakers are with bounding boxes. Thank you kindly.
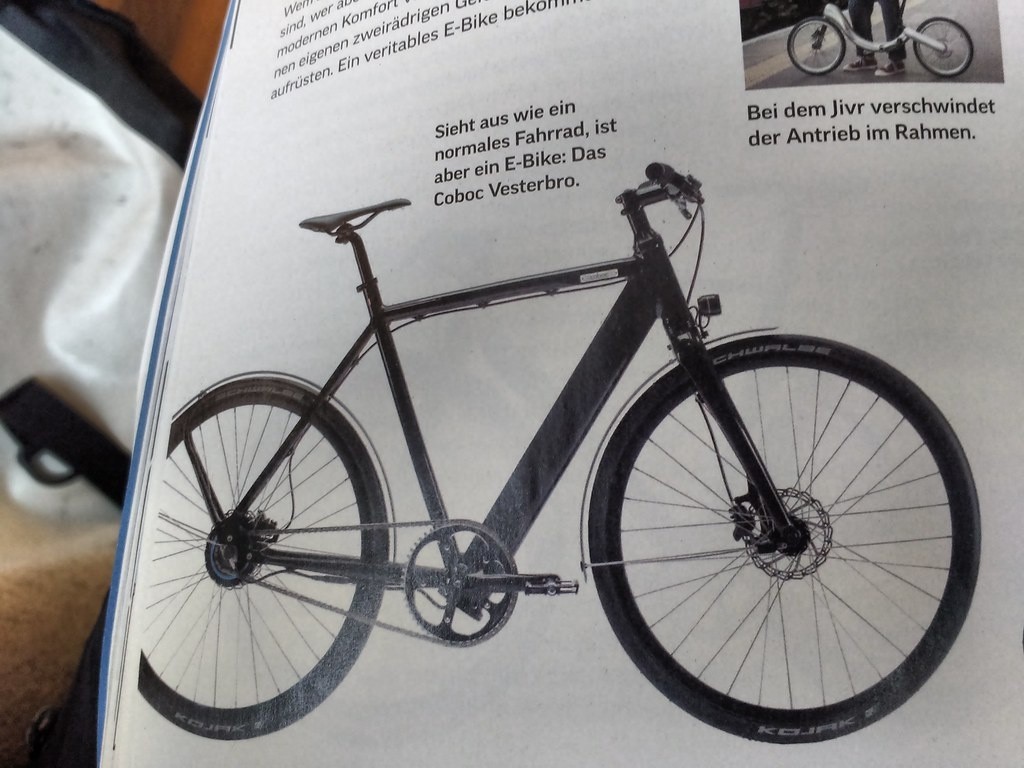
[843,57,879,70]
[875,61,905,76]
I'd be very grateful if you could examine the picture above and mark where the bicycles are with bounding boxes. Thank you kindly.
[786,0,976,77]
[138,158,982,746]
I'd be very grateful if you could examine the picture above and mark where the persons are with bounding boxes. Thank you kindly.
[843,0,907,76]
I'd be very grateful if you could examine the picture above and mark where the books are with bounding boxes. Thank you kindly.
[97,0,1024,768]
